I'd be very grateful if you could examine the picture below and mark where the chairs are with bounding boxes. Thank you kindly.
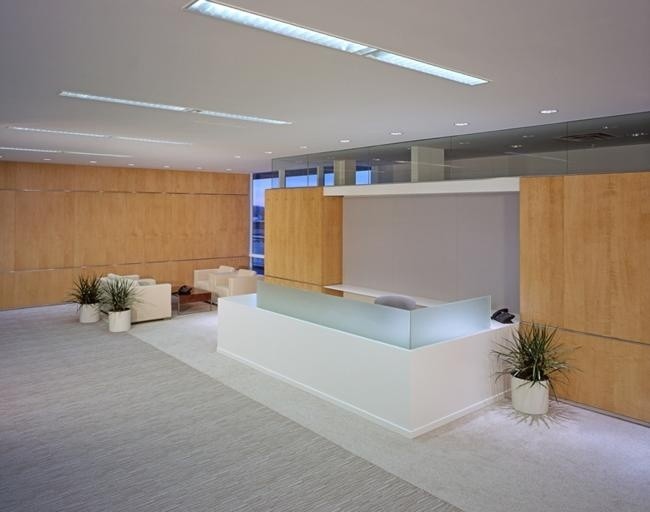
[193,264,257,306]
[96,275,172,324]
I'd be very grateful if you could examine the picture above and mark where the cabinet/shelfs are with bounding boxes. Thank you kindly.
[264,187,343,298]
[516,171,650,425]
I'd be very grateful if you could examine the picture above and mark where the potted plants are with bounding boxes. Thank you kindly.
[489,320,585,415]
[64,272,108,324]
[99,276,144,333]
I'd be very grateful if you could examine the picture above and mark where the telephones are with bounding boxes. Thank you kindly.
[491,309,515,323]
[178,285,192,294]
